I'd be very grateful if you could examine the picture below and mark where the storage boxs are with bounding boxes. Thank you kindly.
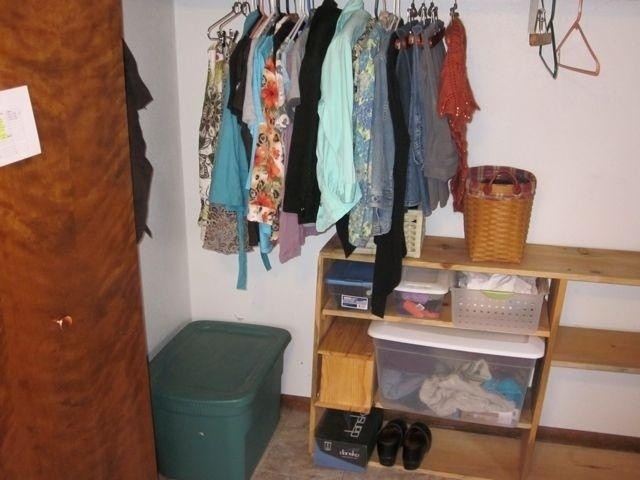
[365,321,547,431]
[150,319,292,480]
[323,258,549,337]
[312,406,384,472]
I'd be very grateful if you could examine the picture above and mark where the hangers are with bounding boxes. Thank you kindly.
[529,1,600,79]
[206,0,467,59]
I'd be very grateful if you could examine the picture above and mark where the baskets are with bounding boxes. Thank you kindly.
[462,164,538,265]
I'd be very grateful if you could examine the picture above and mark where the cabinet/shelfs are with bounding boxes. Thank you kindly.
[0,0,157,480]
[306,232,640,480]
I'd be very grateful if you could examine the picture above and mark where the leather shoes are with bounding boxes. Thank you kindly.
[401,421,432,471]
[376,418,407,467]
